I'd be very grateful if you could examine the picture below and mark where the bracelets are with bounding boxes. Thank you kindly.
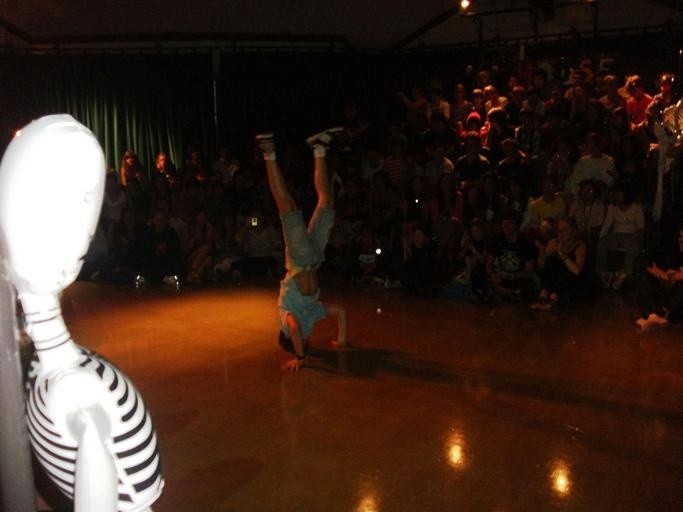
[295,354,308,360]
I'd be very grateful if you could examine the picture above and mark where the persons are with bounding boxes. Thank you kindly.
[256,121,348,373]
[337,50,683,330]
[71,133,337,293]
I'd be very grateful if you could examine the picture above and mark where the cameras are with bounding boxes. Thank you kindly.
[251,217,258,227]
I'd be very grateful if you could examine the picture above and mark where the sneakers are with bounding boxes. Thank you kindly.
[306,127,346,150]
[254,133,275,152]
